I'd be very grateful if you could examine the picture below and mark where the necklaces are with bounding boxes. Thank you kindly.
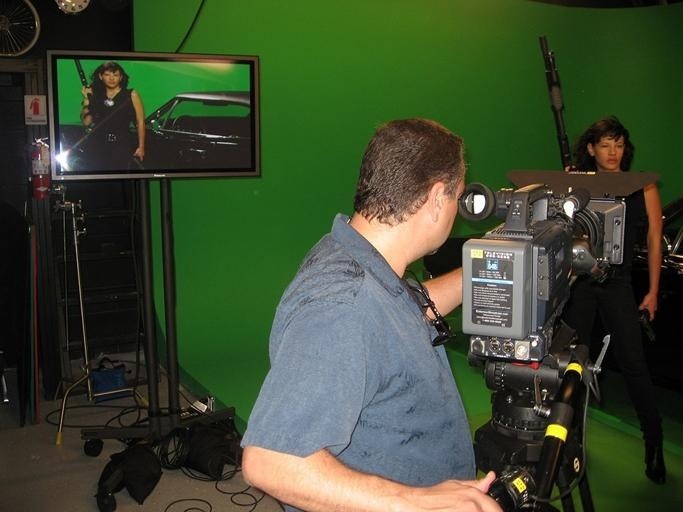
[104,87,120,107]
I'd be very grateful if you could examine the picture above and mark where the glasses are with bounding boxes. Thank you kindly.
[403,269,458,348]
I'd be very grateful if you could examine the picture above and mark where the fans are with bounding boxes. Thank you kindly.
[0,0,40,57]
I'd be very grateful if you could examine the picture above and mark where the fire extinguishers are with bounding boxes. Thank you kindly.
[31,137,50,200]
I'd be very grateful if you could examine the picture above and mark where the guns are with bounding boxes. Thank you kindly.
[538,29,574,173]
[74,59,97,116]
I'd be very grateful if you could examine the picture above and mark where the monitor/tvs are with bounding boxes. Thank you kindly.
[47,50,261,180]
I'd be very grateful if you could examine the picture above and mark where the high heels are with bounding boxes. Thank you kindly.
[643,433,667,487]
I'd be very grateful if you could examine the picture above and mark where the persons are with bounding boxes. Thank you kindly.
[240,119,504,512]
[565,116,666,484]
[81,62,145,171]
